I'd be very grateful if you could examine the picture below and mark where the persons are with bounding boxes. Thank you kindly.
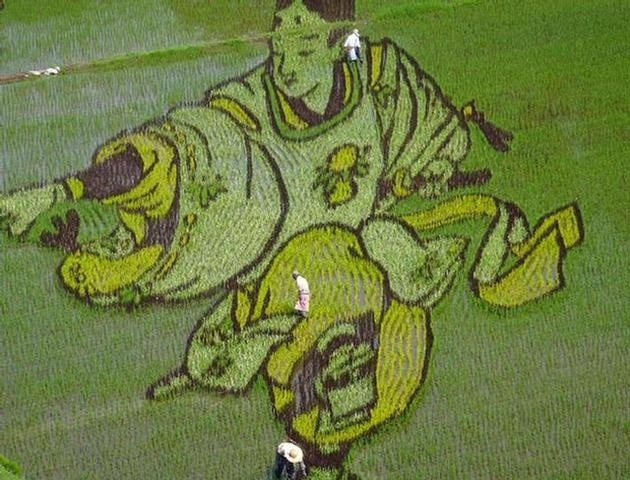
[292,270,309,317]
[344,28,360,61]
[24,66,60,76]
[275,443,307,480]
[1,0,586,480]
